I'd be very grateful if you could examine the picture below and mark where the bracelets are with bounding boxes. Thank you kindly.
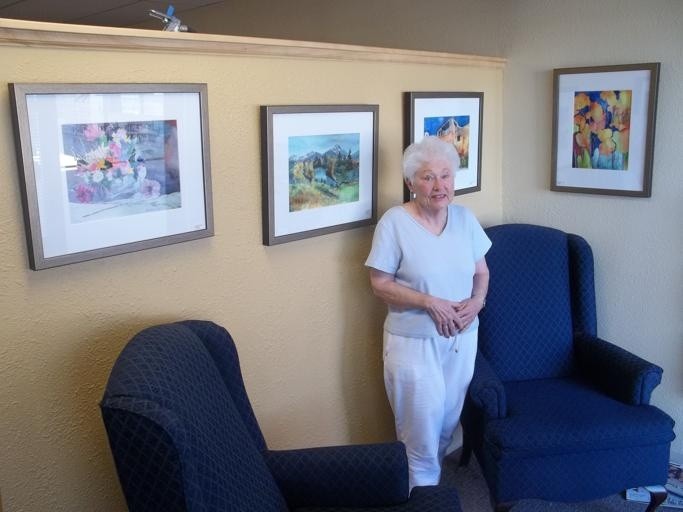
[472,293,486,308]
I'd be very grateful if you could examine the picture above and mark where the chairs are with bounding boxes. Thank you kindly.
[459,224,676,512]
[97,320,459,512]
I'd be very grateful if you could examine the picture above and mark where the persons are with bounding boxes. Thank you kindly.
[361,138,493,498]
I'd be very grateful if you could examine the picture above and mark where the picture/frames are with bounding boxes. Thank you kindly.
[550,62,660,197]
[260,105,378,245]
[404,92,484,203]
[7,83,214,270]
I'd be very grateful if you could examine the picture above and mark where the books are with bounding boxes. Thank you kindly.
[625,461,683,508]
